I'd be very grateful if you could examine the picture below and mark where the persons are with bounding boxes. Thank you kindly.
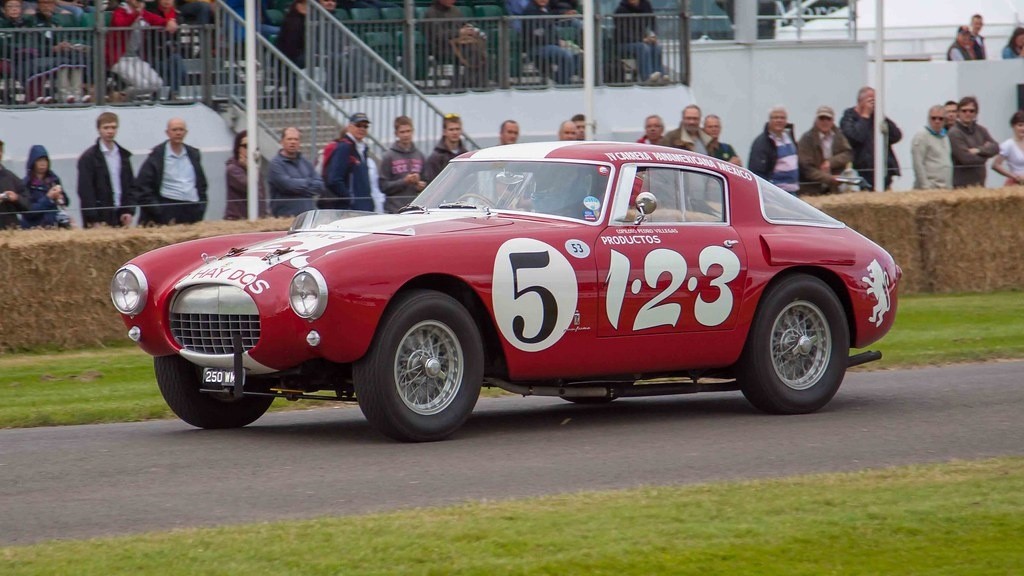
[421,0,487,88]
[499,120,519,145]
[613,0,670,82]
[748,108,801,197]
[76,112,137,227]
[0,139,70,228]
[224,113,375,220]
[1002,27,1024,59]
[558,114,584,140]
[840,86,903,192]
[502,0,583,85]
[0,0,355,104]
[638,105,742,166]
[424,114,467,183]
[379,116,427,213]
[134,118,209,227]
[948,14,986,60]
[991,110,1024,185]
[911,95,999,189]
[798,106,852,196]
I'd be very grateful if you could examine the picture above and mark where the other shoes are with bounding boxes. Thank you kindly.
[646,70,661,86]
[658,72,670,86]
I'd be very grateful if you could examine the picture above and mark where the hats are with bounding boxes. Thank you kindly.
[349,112,372,126]
[816,106,835,119]
[957,25,971,36]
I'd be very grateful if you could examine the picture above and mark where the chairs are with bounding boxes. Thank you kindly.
[0,0,650,106]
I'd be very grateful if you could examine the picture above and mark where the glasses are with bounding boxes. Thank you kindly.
[930,115,945,121]
[959,108,977,113]
[240,142,247,150]
[353,124,369,129]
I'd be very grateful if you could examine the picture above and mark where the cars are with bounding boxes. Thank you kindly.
[110,139,905,442]
